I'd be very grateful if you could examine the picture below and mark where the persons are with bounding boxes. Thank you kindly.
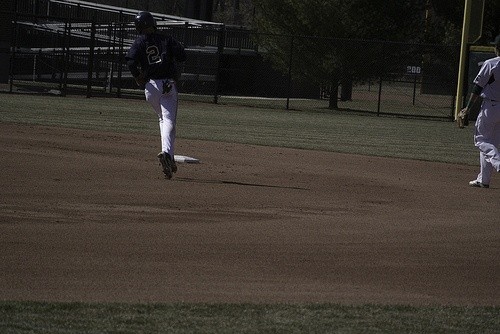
[126,12,184,180]
[459,36,500,187]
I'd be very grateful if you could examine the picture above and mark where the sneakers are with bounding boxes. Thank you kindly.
[156,151,177,180]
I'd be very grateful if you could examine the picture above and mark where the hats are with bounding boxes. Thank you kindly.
[489,35,500,44]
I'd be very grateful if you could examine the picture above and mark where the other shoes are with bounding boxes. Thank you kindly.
[469,179,489,188]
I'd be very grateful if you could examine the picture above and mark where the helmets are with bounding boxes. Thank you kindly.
[134,11,157,34]
